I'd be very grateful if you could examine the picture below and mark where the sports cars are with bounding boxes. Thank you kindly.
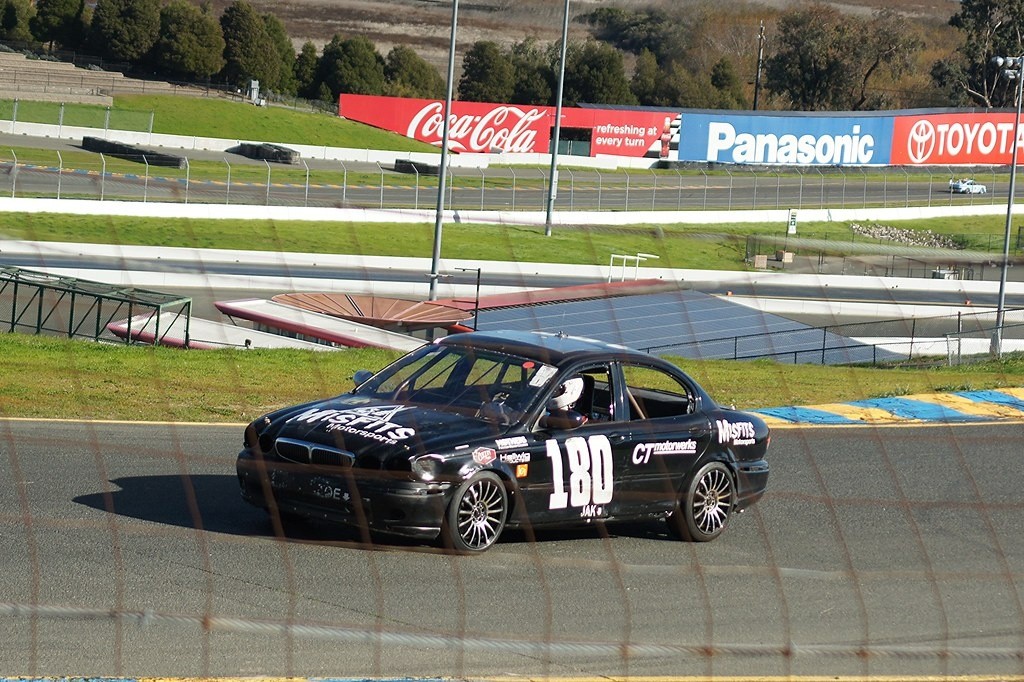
[950,179,987,195]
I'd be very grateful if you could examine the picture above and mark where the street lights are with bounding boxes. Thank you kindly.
[989,55,1024,358]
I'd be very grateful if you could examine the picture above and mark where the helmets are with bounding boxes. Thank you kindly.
[529,366,584,410]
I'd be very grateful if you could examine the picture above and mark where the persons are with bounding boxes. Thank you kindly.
[516,364,591,434]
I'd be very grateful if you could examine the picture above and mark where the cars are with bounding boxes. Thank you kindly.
[236,332,771,556]
